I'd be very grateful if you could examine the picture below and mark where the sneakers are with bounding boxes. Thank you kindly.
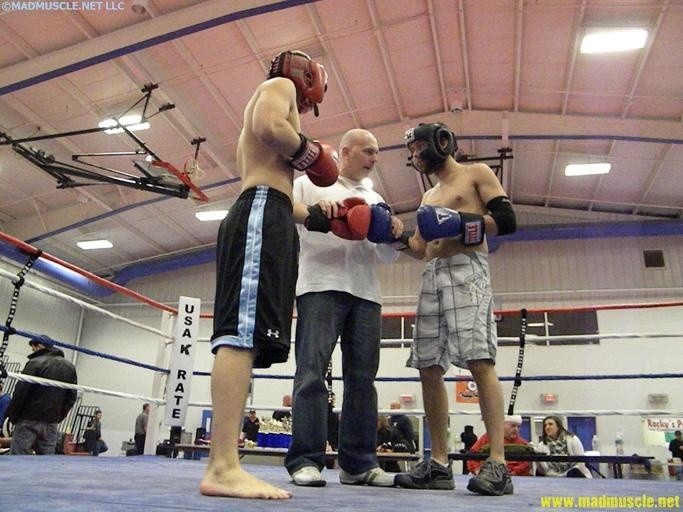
[396,458,454,489]
[467,457,514,495]
[293,466,327,487]
[339,465,398,487]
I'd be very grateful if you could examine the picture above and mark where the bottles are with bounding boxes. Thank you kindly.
[592,434,602,454]
[613,433,624,456]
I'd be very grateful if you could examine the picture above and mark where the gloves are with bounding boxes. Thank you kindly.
[416,203,484,246]
[294,134,342,186]
[306,196,372,242]
[365,202,413,253]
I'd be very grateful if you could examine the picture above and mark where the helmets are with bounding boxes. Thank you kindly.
[268,49,329,113]
[405,120,459,174]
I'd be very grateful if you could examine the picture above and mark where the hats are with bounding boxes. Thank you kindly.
[504,414,523,424]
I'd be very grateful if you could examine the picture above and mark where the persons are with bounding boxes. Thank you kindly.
[0,335,682,478]
[367,121,515,493]
[285,128,405,485]
[198,51,371,501]
